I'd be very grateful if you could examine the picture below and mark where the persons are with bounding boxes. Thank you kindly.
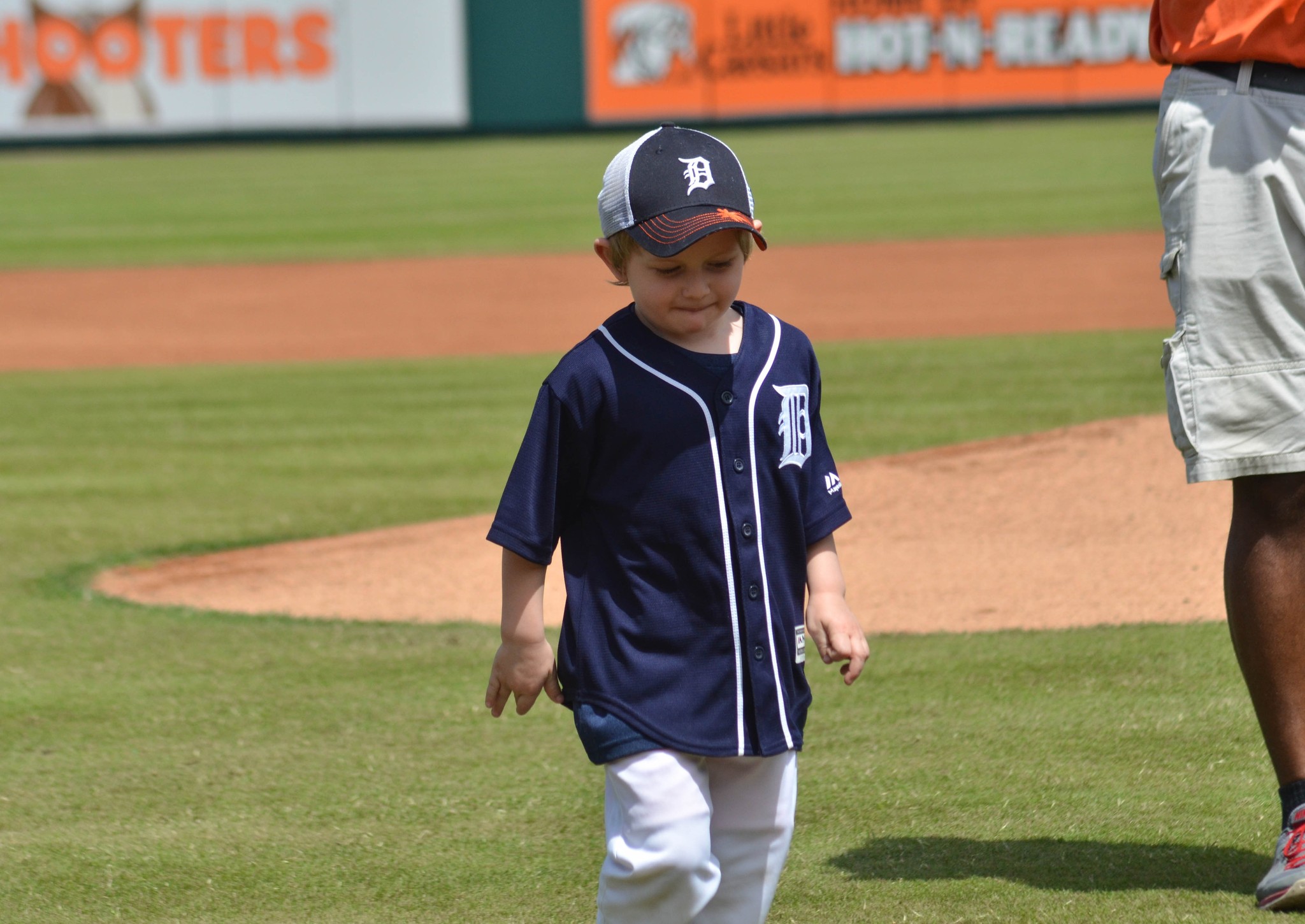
[481,124,870,924]
[1141,1,1305,911]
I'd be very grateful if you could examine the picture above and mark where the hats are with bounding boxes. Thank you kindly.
[596,121,767,257]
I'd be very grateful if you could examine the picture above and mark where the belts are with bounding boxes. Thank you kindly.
[1169,49,1305,99]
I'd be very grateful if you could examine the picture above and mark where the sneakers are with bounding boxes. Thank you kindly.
[1255,802,1305,911]
